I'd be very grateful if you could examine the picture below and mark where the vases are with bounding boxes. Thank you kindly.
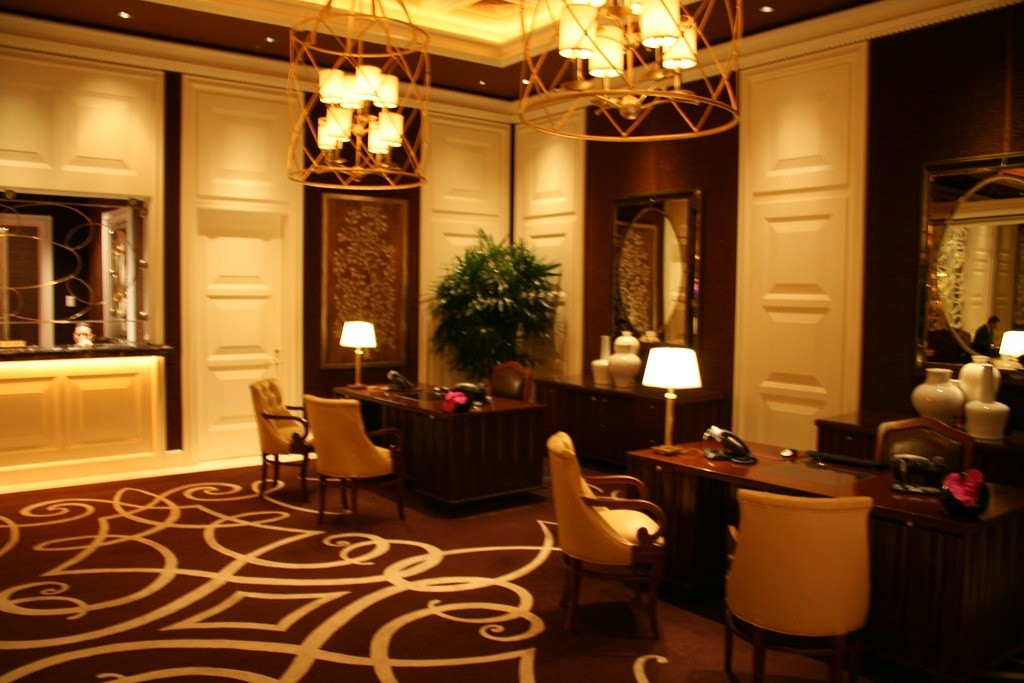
[912,366,966,428]
[965,365,1008,445]
[958,354,1001,400]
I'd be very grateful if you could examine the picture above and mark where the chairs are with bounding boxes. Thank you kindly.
[875,417,975,472]
[546,430,666,640]
[719,489,873,683]
[304,394,404,521]
[490,360,531,401]
[251,378,316,502]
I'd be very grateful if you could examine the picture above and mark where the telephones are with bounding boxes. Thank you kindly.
[385,370,413,392]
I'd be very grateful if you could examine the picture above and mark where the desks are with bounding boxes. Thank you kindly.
[813,410,914,465]
[533,378,718,474]
[336,382,544,517]
[624,438,1024,660]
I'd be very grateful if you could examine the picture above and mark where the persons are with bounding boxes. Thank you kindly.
[74,322,95,343]
[975,315,1000,355]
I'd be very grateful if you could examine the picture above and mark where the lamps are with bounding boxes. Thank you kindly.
[287,0,432,190]
[515,0,744,141]
[339,321,378,390]
[998,331,1024,357]
[641,346,702,455]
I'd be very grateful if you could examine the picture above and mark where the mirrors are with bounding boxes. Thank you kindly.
[913,155,1024,376]
[611,186,704,357]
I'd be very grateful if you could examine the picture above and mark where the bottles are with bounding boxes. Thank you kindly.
[591,336,613,385]
[609,345,642,388]
[959,356,1001,400]
[614,332,640,355]
[639,330,660,343]
[965,364,1010,439]
[912,368,964,424]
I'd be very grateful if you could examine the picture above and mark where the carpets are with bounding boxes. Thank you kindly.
[0,458,1023,683]
